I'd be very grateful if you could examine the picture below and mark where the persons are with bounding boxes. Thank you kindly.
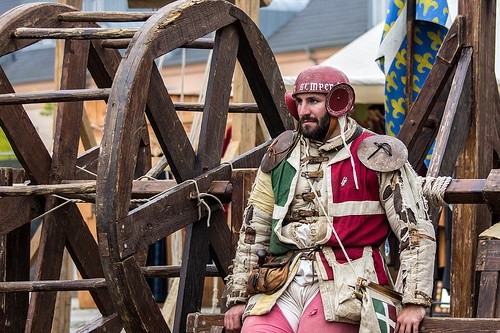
[225,66,436,333]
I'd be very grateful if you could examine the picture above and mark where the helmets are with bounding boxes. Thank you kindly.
[292,66,349,99]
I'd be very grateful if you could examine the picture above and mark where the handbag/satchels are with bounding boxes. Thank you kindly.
[333,256,379,322]
[246,251,295,295]
[355,277,407,333]
